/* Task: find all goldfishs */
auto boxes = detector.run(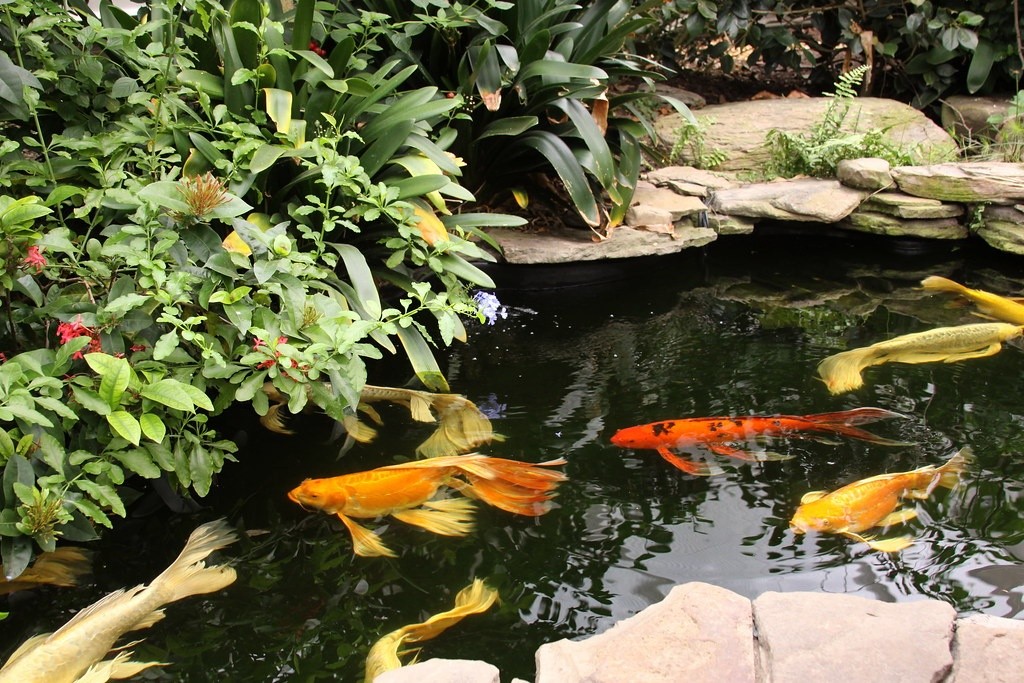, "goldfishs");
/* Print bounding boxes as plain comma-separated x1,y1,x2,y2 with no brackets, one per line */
0,275,1024,683
